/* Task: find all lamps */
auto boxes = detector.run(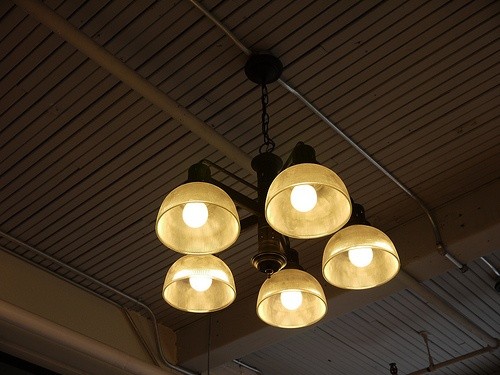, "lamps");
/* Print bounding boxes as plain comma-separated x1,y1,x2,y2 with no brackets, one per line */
155,53,403,329
